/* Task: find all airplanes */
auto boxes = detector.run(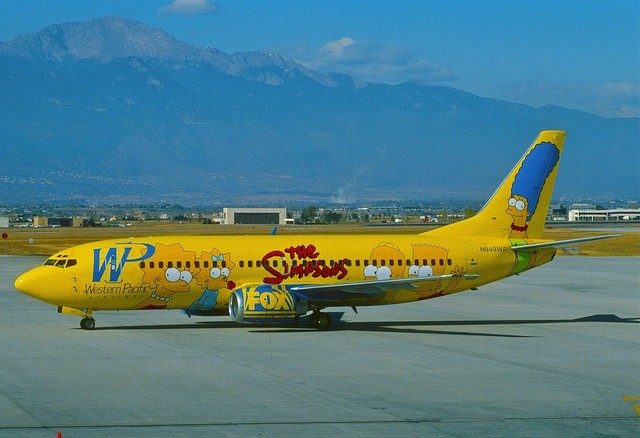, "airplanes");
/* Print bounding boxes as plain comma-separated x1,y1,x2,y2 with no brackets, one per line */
15,132,624,330
